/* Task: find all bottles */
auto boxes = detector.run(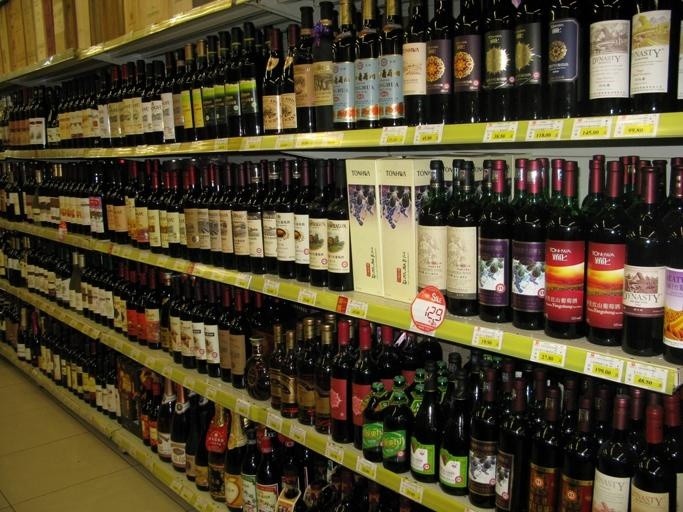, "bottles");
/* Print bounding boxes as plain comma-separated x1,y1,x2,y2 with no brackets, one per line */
0,159,683,367
0,0,683,148
40,367,203,493
202,367,682,511
